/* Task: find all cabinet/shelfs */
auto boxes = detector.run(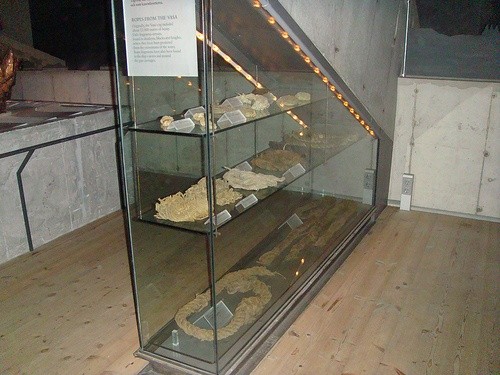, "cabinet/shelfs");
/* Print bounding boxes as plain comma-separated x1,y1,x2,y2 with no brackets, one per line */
119,89,381,375
